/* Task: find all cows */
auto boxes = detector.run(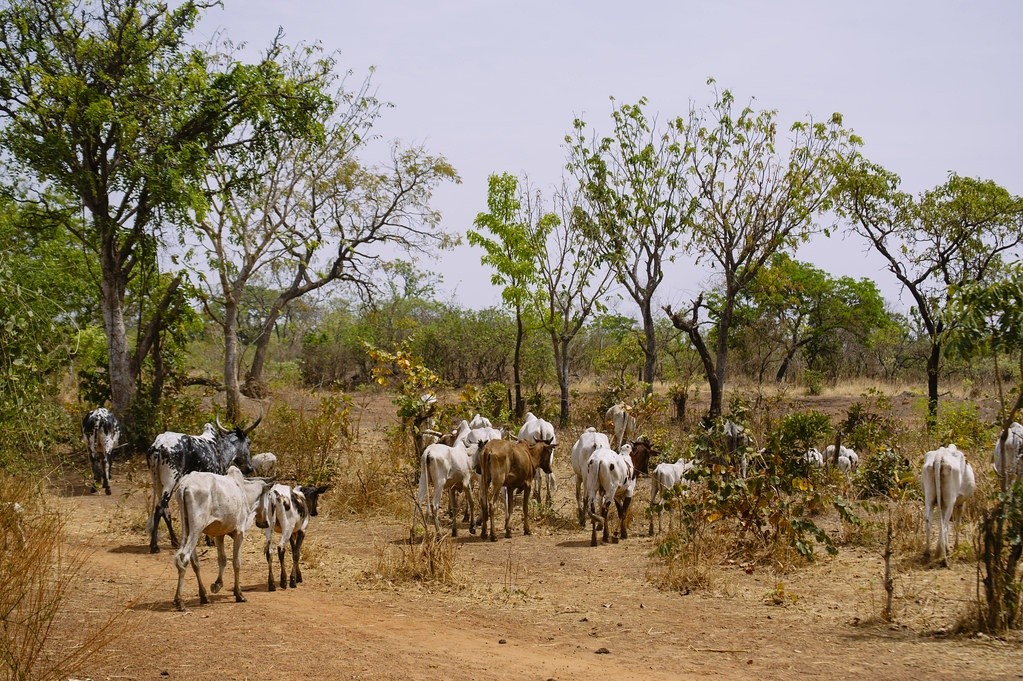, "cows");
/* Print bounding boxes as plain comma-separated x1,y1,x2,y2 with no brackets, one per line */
801,444,860,475
570,401,661,548
146,403,332,613
919,443,977,568
648,457,703,537
409,412,560,543
82,407,122,496
992,421,1023,496
